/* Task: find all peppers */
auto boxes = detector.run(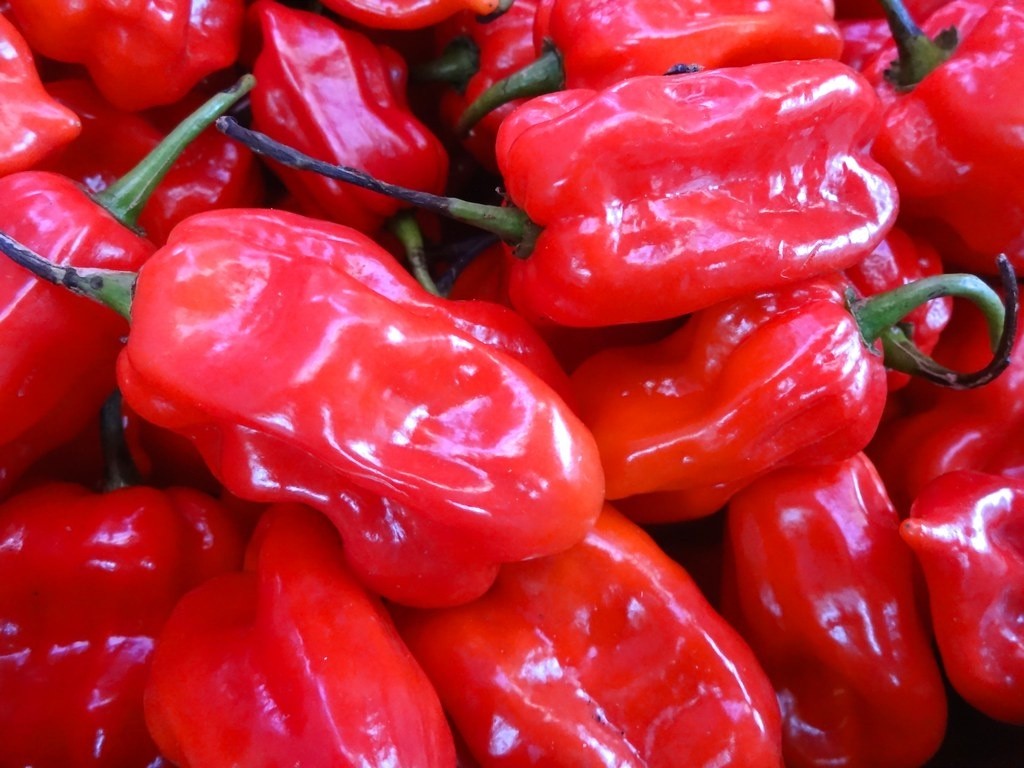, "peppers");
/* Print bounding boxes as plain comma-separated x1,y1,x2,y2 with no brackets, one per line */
0,0,1024,768
